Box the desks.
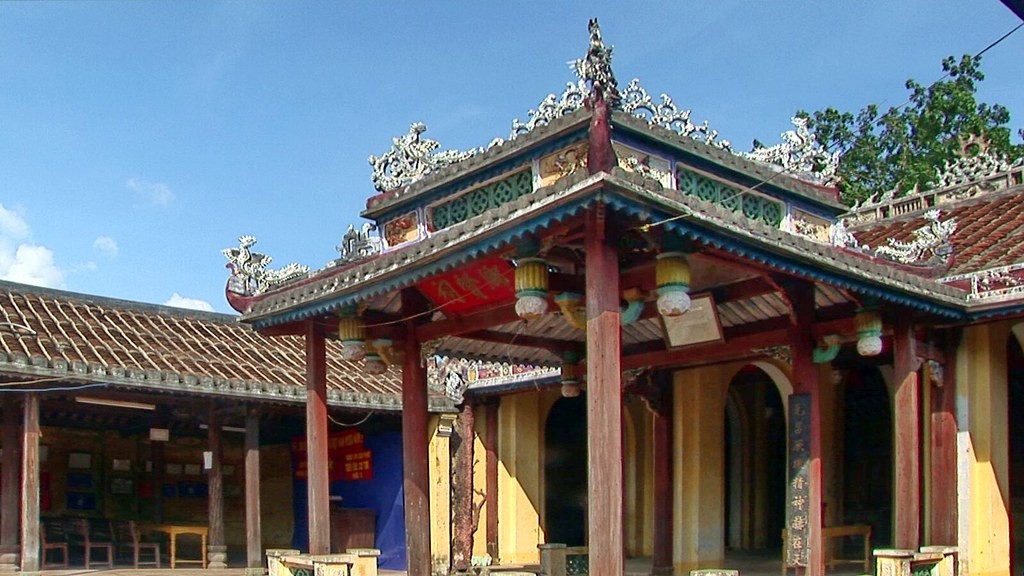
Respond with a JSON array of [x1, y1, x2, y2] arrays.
[[781, 525, 871, 576], [141, 523, 208, 569]]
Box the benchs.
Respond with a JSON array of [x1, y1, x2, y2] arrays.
[[40, 515, 160, 569]]
